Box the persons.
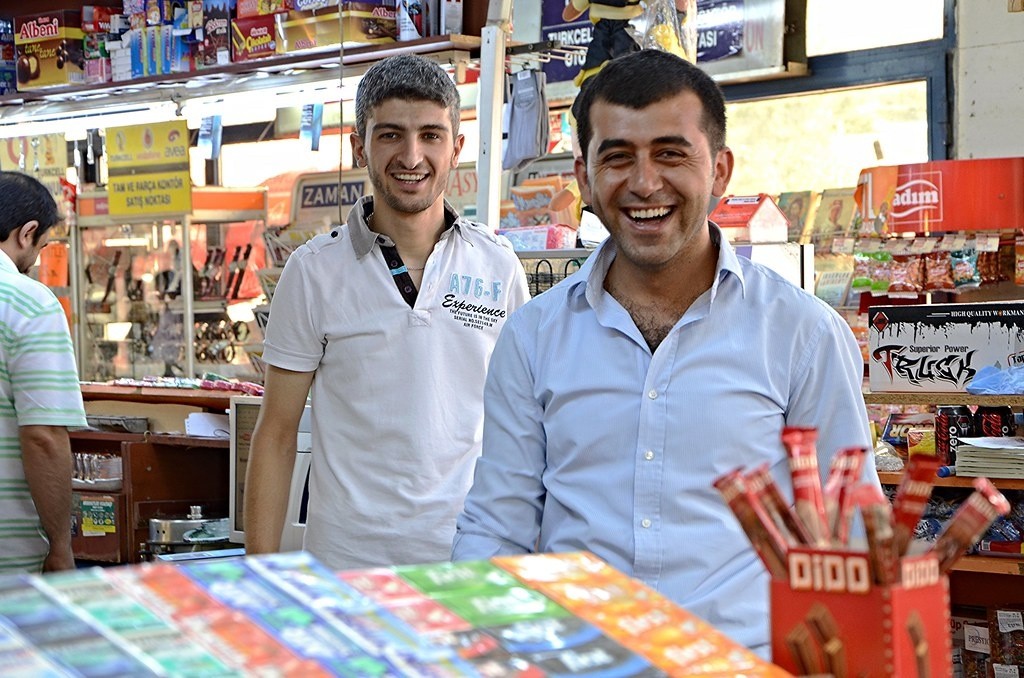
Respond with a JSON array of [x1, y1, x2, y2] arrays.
[[242, 51, 531, 571], [450, 48, 894, 660], [0, 171, 87, 574]]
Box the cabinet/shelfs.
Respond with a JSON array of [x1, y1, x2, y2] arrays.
[[506, 243, 814, 303], [65, 430, 224, 565], [0, 36, 530, 434], [849, 159, 1023, 677]]
